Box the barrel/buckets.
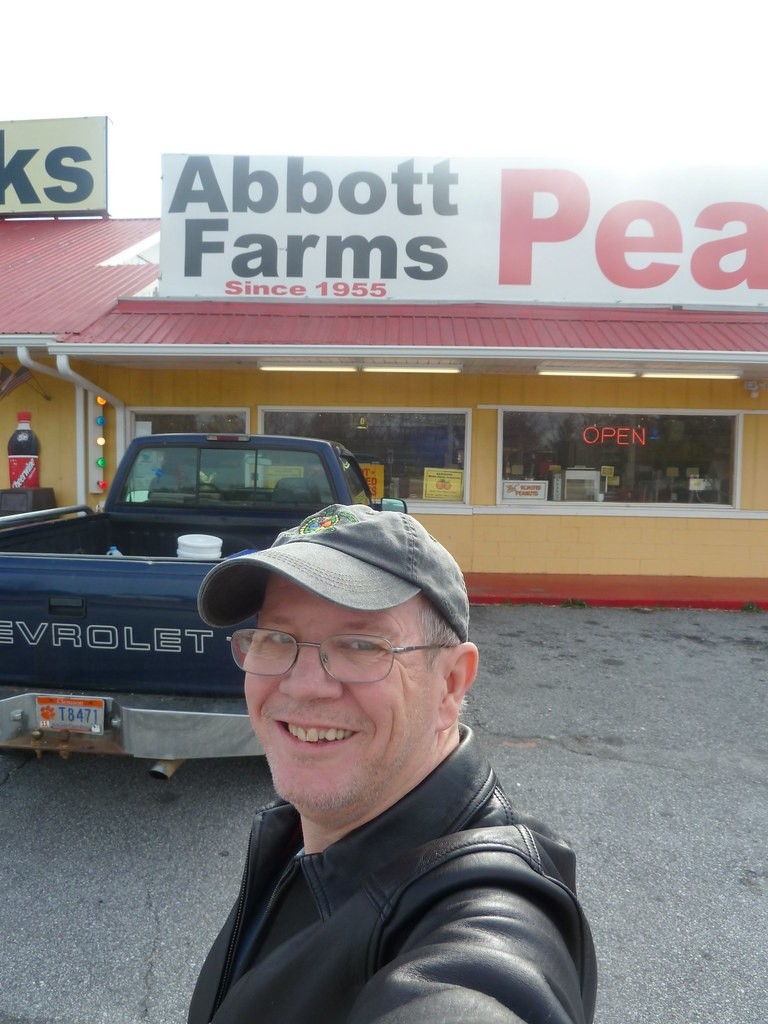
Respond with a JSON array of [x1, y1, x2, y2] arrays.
[[176, 534, 223, 558]]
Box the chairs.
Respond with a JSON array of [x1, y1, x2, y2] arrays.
[[143, 476, 193, 503], [271, 477, 322, 504]]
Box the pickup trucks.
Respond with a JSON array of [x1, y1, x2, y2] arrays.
[[0, 432, 409, 782]]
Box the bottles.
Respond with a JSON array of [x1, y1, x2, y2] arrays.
[[8, 412, 40, 488], [106, 546, 123, 556]]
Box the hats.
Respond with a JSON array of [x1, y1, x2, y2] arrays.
[[196, 503, 470, 644]]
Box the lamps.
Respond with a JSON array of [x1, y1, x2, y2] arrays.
[[743, 380, 760, 398]]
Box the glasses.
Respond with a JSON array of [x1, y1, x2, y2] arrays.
[[230, 626, 459, 684]]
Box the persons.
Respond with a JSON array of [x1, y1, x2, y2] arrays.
[[187, 504, 598, 1024]]
